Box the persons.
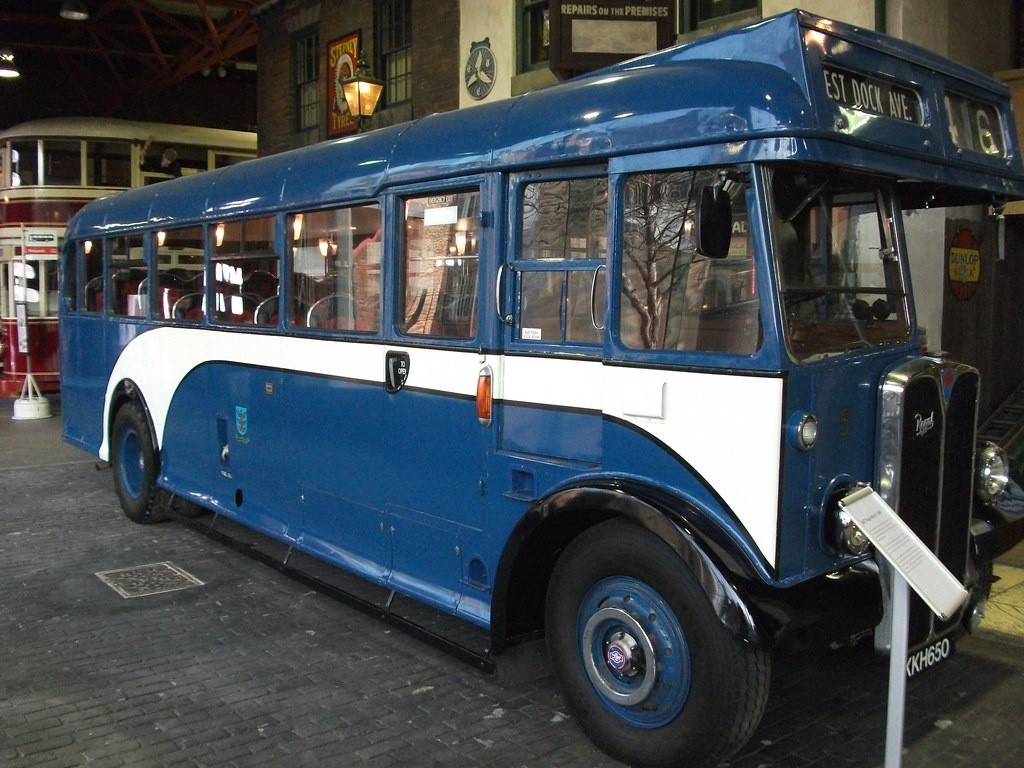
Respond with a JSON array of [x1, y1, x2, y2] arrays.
[[336, 63, 351, 100], [139, 149, 184, 185]]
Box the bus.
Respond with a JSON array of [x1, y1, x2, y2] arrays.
[[58, 8, 1022, 766], [0, 114, 257, 394]]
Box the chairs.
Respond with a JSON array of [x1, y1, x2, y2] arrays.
[[87, 264, 478, 340]]
